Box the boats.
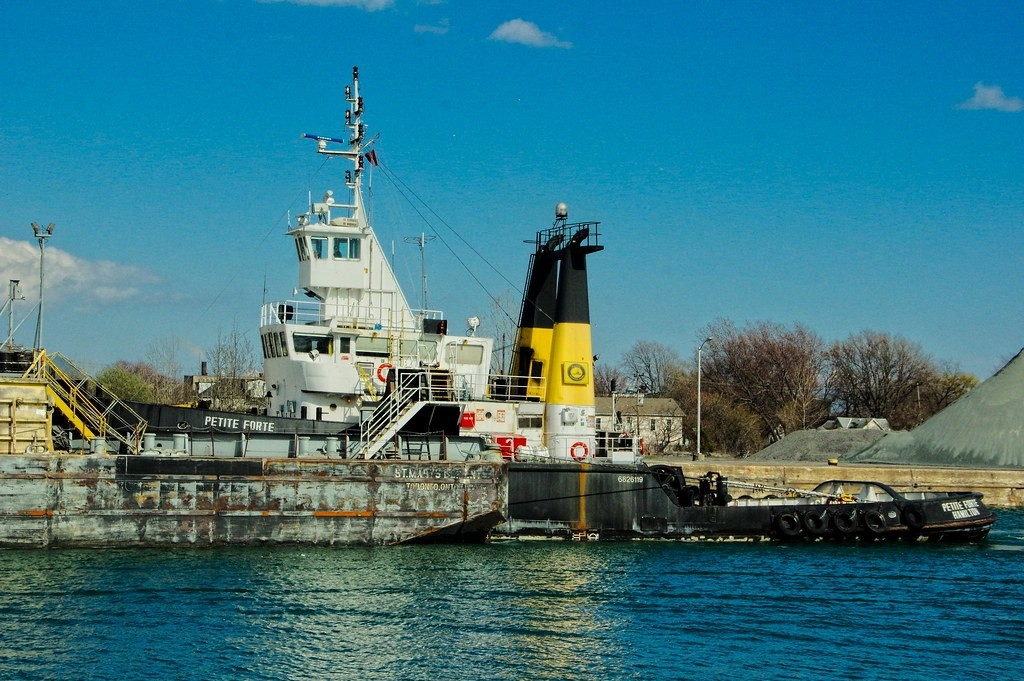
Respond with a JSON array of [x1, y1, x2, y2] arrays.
[[1, 67, 998, 547]]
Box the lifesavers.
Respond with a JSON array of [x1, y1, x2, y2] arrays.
[[377, 363, 393, 383], [773, 501, 928, 539], [570, 442, 588, 462], [640, 437, 645, 456]]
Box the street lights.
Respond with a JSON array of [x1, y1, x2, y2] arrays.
[[693, 337, 712, 460]]
